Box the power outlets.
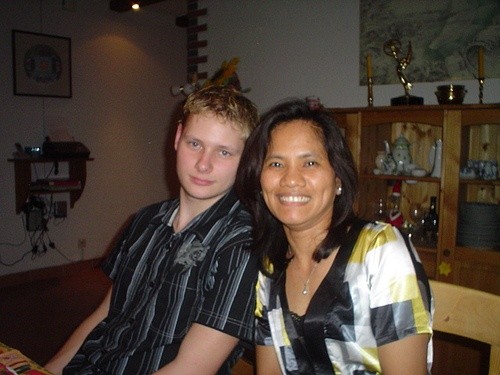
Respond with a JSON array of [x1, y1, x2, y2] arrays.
[[79, 239, 87, 247]]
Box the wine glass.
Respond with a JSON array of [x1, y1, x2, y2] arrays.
[[409, 203, 423, 226]]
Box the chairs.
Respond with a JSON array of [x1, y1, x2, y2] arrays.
[[429, 280, 500, 375]]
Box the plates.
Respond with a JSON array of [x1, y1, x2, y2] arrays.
[[457, 202, 500, 248]]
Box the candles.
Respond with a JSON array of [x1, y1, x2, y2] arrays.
[[479, 47, 484, 78], [367, 55, 372, 78]]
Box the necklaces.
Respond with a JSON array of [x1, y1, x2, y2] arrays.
[[291, 261, 319, 296]]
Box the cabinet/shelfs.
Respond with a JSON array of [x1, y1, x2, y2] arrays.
[[326, 103, 500, 375], [8, 158, 97, 214]]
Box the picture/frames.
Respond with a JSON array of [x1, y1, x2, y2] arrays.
[[12, 28, 72, 99]]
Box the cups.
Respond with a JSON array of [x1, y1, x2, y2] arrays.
[[460, 159, 498, 180], [373, 141, 427, 177]]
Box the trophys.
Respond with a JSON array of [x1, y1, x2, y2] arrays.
[[384, 38, 424, 106]]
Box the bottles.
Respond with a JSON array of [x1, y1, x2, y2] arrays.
[[375, 197, 388, 223], [423, 196, 438, 245], [431, 139, 441, 178]]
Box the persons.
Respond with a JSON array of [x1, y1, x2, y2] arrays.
[[239, 96, 435, 375], [45, 84, 256, 375]]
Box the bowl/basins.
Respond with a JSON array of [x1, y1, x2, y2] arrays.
[[435, 85, 468, 104]]
